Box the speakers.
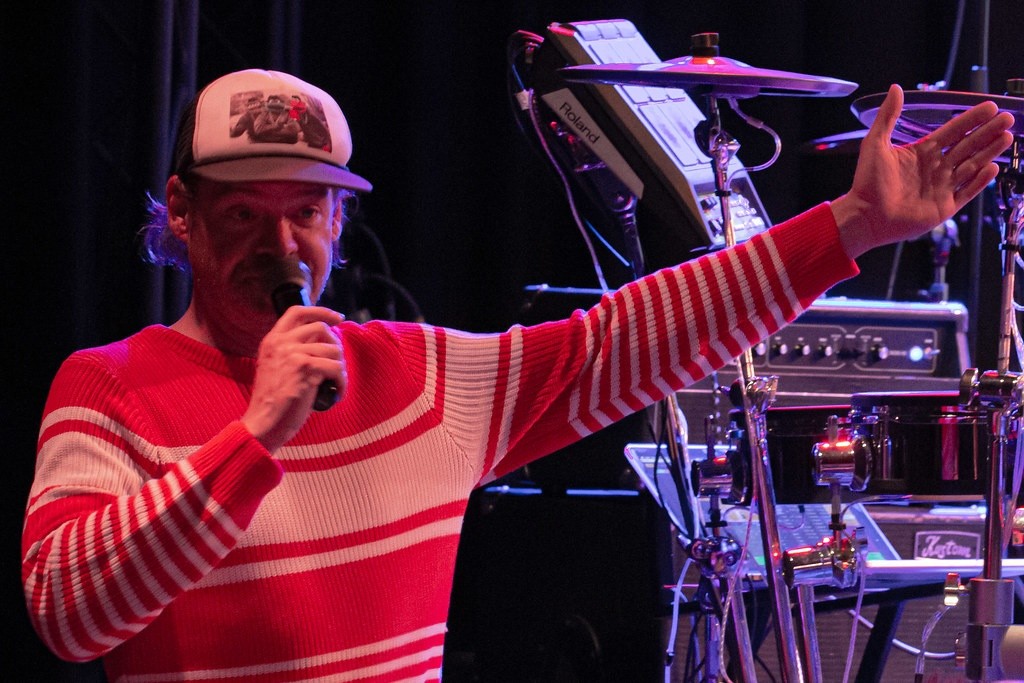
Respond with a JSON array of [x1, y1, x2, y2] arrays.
[[442, 484, 668, 683]]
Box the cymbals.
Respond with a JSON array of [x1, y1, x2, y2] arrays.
[[849, 91, 1024, 164], [549, 56, 860, 99], [797, 128, 869, 155]]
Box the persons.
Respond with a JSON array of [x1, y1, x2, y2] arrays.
[[21, 69, 1015, 683]]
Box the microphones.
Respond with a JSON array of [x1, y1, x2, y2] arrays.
[[264, 257, 338, 413]]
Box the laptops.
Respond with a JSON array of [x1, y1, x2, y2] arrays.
[[626, 440, 1024, 580]]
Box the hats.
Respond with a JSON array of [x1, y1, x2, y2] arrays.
[[181, 67, 373, 192]]
